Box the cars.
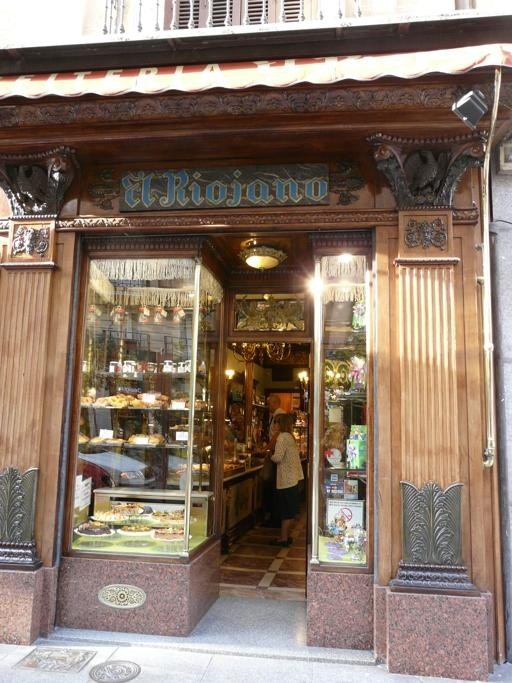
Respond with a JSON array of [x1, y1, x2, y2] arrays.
[[79, 434, 159, 490]]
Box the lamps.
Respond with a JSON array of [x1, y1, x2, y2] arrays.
[[448, 88, 488, 129], [238, 246, 288, 273], [65, 250, 231, 563]]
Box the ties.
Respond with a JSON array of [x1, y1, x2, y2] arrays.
[[269, 415, 274, 427]]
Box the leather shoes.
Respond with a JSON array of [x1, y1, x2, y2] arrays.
[[259, 520, 281, 528], [288, 537, 292, 544], [270, 539, 289, 548]]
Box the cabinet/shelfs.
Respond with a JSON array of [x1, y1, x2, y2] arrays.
[[167, 457, 307, 549], [311, 249, 374, 574]]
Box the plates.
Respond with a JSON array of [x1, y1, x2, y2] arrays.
[[73, 528, 115, 538], [128, 405, 160, 409], [88, 442, 124, 447], [123, 442, 159, 448], [153, 536, 191, 543], [116, 529, 153, 536], [166, 406, 197, 411], [90, 515, 129, 525], [148, 513, 195, 526], [92, 403, 123, 409]]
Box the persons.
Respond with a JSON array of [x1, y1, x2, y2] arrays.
[[258, 395, 287, 453], [270, 415, 305, 546], [254, 413, 284, 528]]
